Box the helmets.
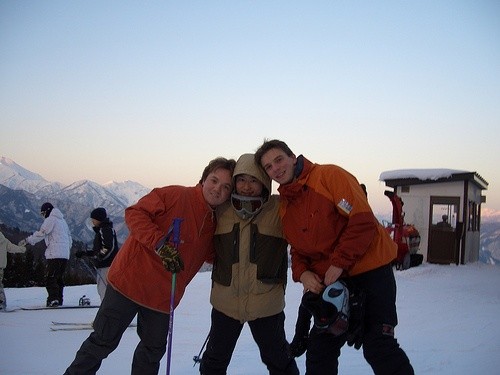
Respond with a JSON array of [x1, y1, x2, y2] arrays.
[[302, 279, 350, 331], [41, 203, 54, 212]]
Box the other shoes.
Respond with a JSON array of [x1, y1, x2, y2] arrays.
[[45, 300, 59, 307]]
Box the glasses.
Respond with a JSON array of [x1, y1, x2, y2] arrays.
[[230, 193, 263, 215]]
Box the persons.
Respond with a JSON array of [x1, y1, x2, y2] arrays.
[[65, 159, 237, 375], [255, 139, 415, 375], [18, 203, 72, 306], [0, 232, 26, 310], [437, 214, 451, 226], [197, 154, 301, 375], [76, 208, 119, 301]]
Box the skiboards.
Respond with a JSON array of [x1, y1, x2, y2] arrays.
[[16, 304, 101, 311], [49, 320, 138, 331]]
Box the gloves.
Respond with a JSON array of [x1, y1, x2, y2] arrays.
[[346, 293, 370, 349], [75, 251, 84, 259], [18, 239, 27, 247], [91, 255, 99, 265], [289, 323, 309, 356], [155, 245, 184, 273]]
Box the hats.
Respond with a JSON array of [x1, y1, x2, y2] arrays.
[[91, 207, 106, 221]]
